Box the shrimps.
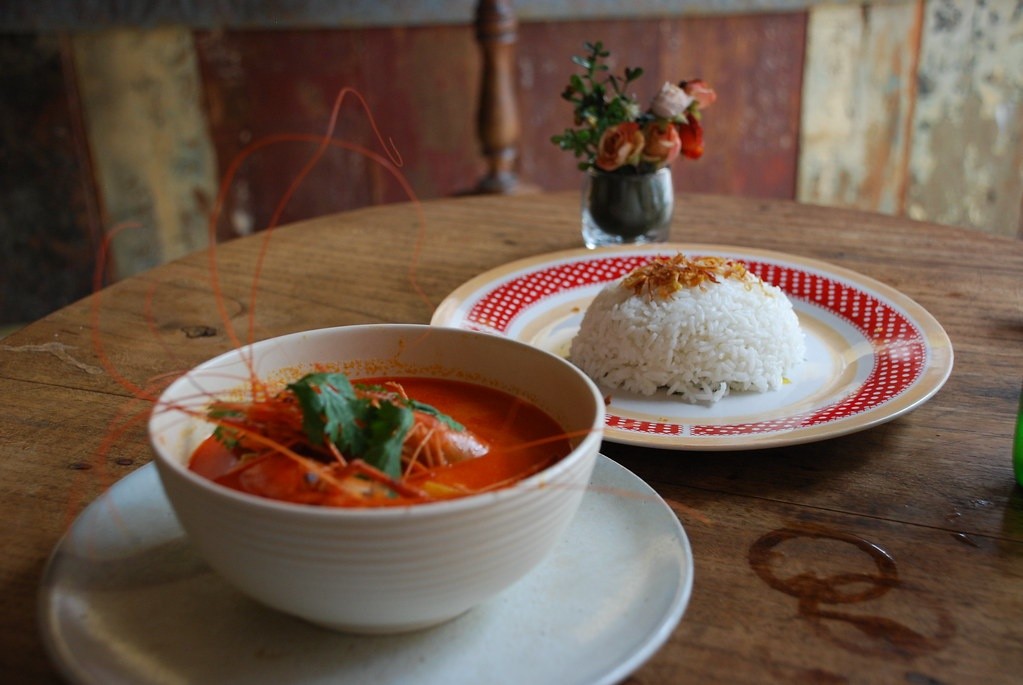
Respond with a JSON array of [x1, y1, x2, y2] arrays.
[[234, 384, 499, 507]]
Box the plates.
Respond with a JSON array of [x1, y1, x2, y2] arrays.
[[34, 434, 695, 685], [425, 241, 955, 451]]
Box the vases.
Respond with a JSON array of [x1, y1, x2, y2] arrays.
[[579, 166, 675, 251]]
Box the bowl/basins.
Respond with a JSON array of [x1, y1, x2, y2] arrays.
[[151, 322, 605, 635]]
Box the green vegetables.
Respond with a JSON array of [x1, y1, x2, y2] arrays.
[[208, 371, 466, 497]]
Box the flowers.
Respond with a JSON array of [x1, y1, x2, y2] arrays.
[[547, 38, 714, 231]]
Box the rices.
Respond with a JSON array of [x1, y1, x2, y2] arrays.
[[568, 248, 809, 406]]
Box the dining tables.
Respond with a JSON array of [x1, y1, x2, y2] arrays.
[[0, 184, 1022, 685]]
